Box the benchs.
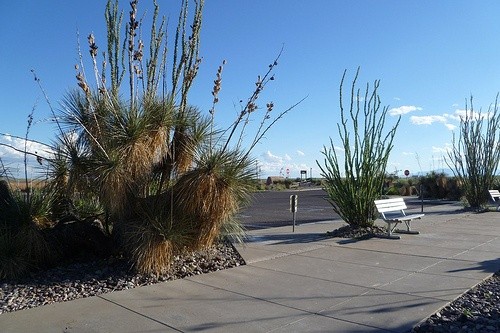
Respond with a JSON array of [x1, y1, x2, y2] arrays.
[[488, 189, 500, 210], [374, 197, 425, 239]]
